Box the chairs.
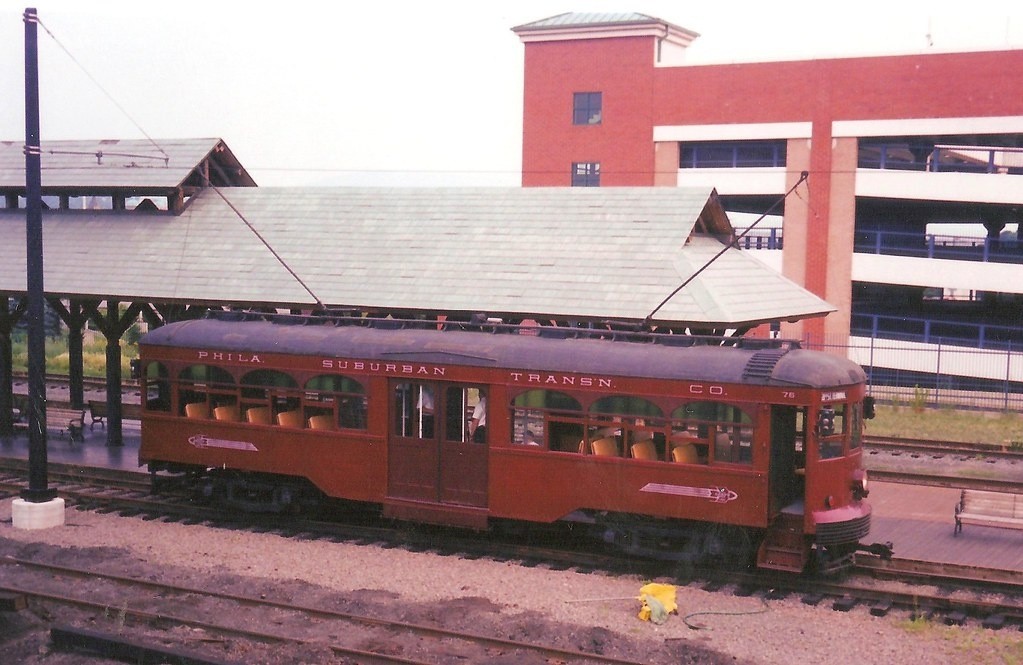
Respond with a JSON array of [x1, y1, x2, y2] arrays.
[[184, 402, 336, 428], [564, 426, 731, 464]]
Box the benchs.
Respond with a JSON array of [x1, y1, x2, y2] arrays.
[[12, 393, 30, 424], [87, 400, 142, 430], [953, 487, 1023, 535]]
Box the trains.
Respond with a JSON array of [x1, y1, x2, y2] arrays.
[[136, 309, 897, 587]]
